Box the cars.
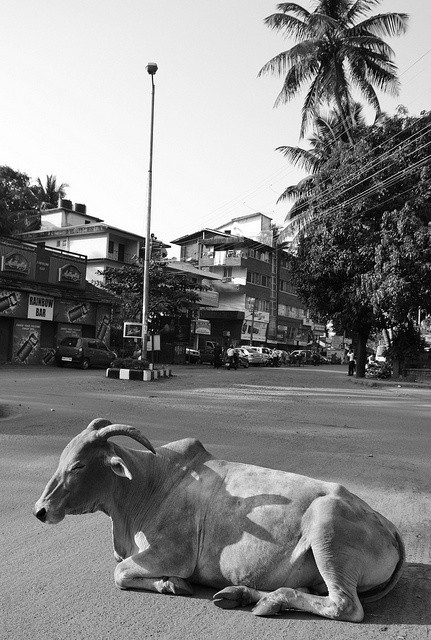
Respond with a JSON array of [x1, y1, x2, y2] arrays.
[[232, 350, 248, 367], [235, 348, 263, 366]]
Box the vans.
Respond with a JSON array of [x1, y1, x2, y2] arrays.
[[290, 350, 313, 358], [241, 346, 278, 363], [56, 336, 117, 369]]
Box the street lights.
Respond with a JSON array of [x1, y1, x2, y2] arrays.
[[141, 62, 158, 361]]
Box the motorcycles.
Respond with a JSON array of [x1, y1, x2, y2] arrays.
[[265, 357, 281, 367], [224, 355, 239, 370]]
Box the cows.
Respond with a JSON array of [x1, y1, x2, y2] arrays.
[[30, 416, 406, 624]]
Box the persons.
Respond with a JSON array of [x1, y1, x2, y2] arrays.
[[272, 348, 279, 366], [366, 355, 374, 368], [227, 344, 238, 366], [282, 351, 287, 365], [348, 349, 355, 376], [214, 343, 222, 368], [133, 344, 143, 360]]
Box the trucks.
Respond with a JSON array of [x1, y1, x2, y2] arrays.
[[196, 341, 223, 364]]
